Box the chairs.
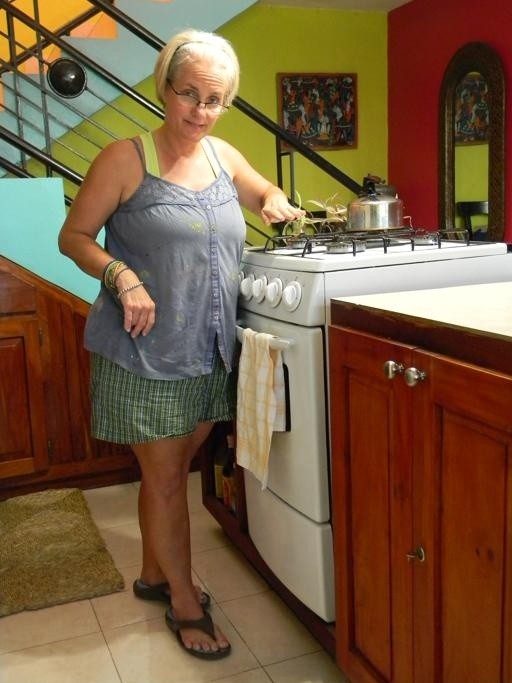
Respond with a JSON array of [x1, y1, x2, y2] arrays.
[[456, 201, 488, 241]]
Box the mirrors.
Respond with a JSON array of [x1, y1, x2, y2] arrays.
[[438, 40, 505, 243]]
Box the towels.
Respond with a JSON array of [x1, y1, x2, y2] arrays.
[[235, 328, 285, 489]]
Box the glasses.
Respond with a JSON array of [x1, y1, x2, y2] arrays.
[[166, 80, 232, 115]]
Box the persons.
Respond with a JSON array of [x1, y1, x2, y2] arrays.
[[53, 24, 309, 660]]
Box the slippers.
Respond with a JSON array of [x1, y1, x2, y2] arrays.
[[133, 576, 214, 608], [162, 602, 231, 661]]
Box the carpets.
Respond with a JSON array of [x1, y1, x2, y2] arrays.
[[0, 487, 125, 616]]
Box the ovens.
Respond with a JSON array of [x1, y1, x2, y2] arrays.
[[229, 309, 332, 626]]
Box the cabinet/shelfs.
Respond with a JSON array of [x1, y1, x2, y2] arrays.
[[0, 256, 144, 487], [201, 418, 247, 547], [327, 325, 512, 683]]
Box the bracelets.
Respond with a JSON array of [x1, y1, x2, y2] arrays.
[[117, 280, 148, 300], [101, 258, 130, 294]]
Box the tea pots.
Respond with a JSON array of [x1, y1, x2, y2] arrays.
[[347, 177, 405, 231]]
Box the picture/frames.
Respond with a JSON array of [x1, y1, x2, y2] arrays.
[[275, 72, 358, 152]]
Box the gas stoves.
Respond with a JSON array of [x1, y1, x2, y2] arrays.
[[235, 228, 509, 325]]
